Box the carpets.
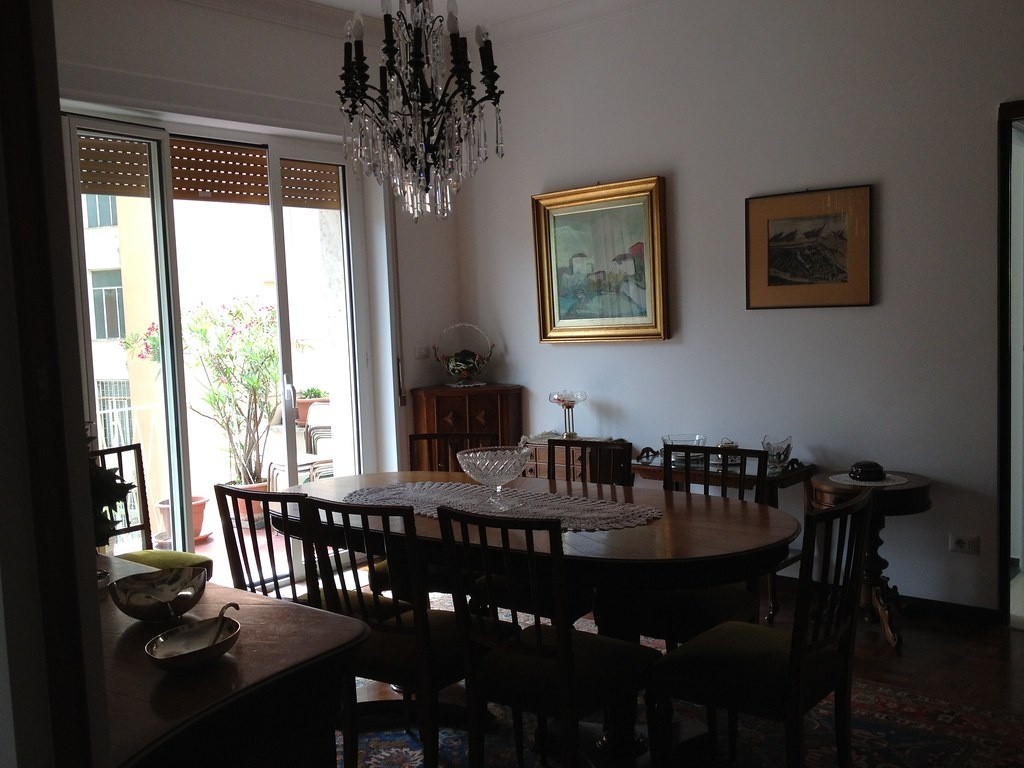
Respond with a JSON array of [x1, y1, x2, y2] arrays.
[[342, 677, 1024, 768]]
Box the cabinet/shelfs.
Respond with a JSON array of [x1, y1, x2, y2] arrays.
[[95, 554, 374, 768], [409, 381, 523, 470], [519, 442, 617, 483]]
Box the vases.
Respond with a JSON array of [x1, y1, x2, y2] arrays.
[[155, 495, 210, 538], [225, 476, 271, 515]]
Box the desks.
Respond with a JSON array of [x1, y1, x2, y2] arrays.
[[809, 470, 933, 648], [633, 453, 818, 510], [276, 471, 801, 768]]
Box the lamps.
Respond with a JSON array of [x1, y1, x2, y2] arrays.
[[334, 0, 505, 222]]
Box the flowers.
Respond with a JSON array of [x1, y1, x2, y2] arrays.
[[117, 292, 283, 484]]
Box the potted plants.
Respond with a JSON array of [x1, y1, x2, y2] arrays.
[[297, 386, 329, 422]]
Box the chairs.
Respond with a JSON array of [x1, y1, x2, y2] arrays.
[[215, 439, 884, 768]]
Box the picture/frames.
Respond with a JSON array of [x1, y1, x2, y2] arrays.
[[744, 183, 874, 310], [531, 175, 672, 344]]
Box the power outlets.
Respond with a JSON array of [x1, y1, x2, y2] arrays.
[[948, 534, 980, 556]]
